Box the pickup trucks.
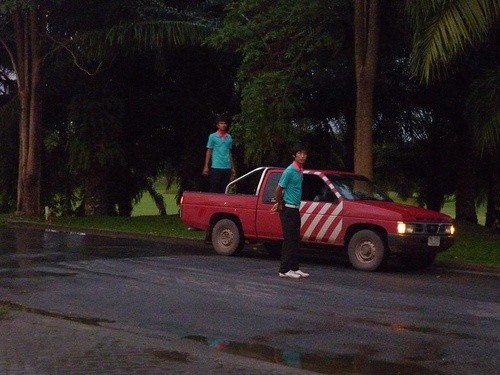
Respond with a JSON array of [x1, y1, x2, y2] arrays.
[[179, 167, 459, 273]]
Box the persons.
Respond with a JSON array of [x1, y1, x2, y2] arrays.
[[274, 145, 311, 279], [202, 116, 236, 193]]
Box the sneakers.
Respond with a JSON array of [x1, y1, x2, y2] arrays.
[[278, 269, 301, 278], [294, 270, 309, 277]]
